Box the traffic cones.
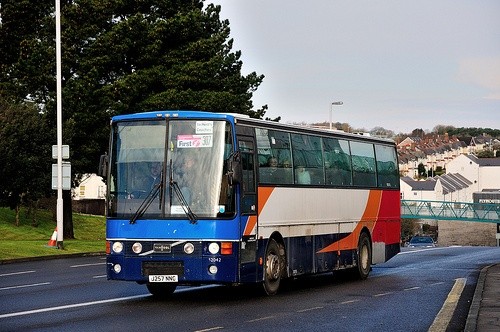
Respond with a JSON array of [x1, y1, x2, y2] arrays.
[[47, 227, 60, 249]]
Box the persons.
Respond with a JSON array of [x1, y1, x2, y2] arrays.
[[259, 156, 346, 185], [127, 162, 161, 200]]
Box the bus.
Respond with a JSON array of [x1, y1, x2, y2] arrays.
[[106, 110, 401, 300]]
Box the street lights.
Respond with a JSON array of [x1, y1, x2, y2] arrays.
[[330, 101, 344, 130]]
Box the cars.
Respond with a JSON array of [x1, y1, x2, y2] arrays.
[[406, 236, 435, 248]]
